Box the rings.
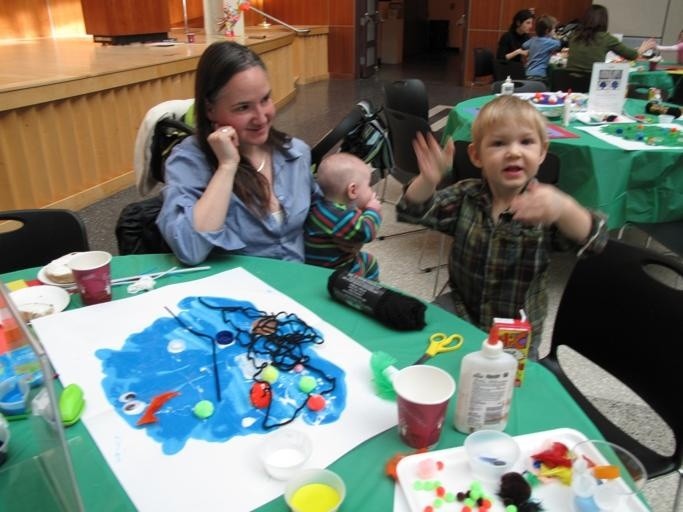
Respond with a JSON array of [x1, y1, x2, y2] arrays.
[[221, 128, 229, 133]]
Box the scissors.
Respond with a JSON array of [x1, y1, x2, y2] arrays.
[[413, 332, 464, 366]]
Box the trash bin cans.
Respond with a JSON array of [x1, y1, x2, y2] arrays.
[[428, 19, 450, 53]]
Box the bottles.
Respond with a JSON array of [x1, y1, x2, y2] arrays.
[[449, 328, 516, 433], [562, 90, 572, 127], [647, 87, 661, 103], [500, 77, 514, 95]]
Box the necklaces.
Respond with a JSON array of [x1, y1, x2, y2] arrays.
[[256, 146, 267, 175]]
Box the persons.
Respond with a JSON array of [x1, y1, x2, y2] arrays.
[[156, 40, 324, 267], [395, 95, 612, 363], [493, 3, 683, 93], [303, 152, 383, 284]]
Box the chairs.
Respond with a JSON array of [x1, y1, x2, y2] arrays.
[[114, 192, 164, 255], [536, 237, 683, 512], [0, 208, 89, 279]]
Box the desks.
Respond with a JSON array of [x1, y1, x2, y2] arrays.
[[386, 40, 683, 244], [0, 256, 646, 512]]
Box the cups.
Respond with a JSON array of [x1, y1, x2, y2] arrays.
[[658, 114, 674, 129], [283, 464, 344, 512], [69, 251, 112, 307], [647, 59, 655, 71], [185, 32, 195, 43], [463, 427, 520, 477], [394, 362, 454, 449], [567, 441, 648, 511], [0, 374, 26, 464]]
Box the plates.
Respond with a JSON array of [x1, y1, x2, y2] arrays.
[[9, 286, 70, 325], [36, 252, 73, 288], [529, 91, 563, 108]]
